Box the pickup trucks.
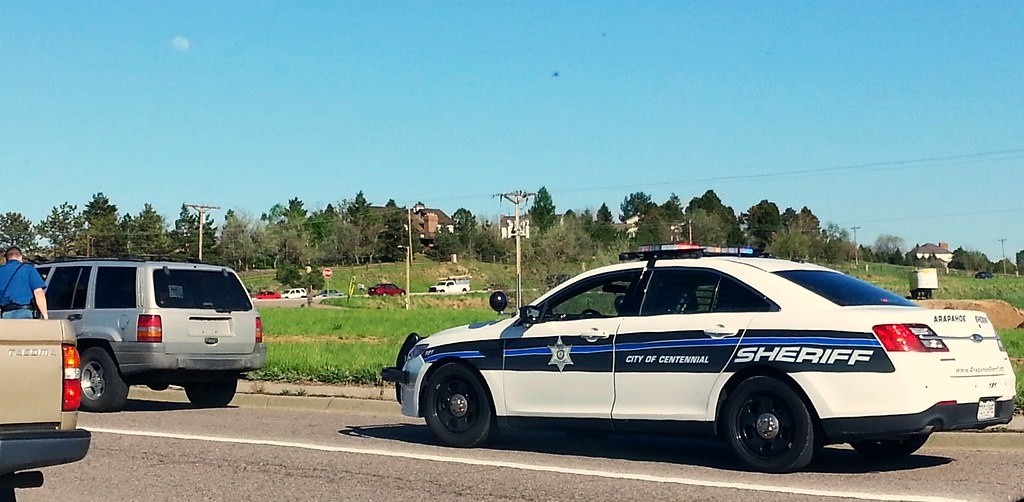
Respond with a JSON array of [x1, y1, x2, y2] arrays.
[[0, 317, 92, 501]]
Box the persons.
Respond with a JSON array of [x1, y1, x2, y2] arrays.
[[0, 248, 51, 320]]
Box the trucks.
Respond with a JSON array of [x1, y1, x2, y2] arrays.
[[430, 276, 470, 294]]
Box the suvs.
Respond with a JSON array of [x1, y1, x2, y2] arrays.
[[30, 259, 266, 409]]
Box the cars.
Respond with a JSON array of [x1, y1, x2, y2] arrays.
[[383, 243, 1017, 475], [250, 290, 281, 299], [282, 287, 309, 298], [975, 270, 993, 279], [368, 283, 406, 296], [544, 274, 571, 289], [483, 283, 509, 290], [316, 288, 344, 300]]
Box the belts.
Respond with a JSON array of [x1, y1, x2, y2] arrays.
[[1, 304, 33, 312]]
